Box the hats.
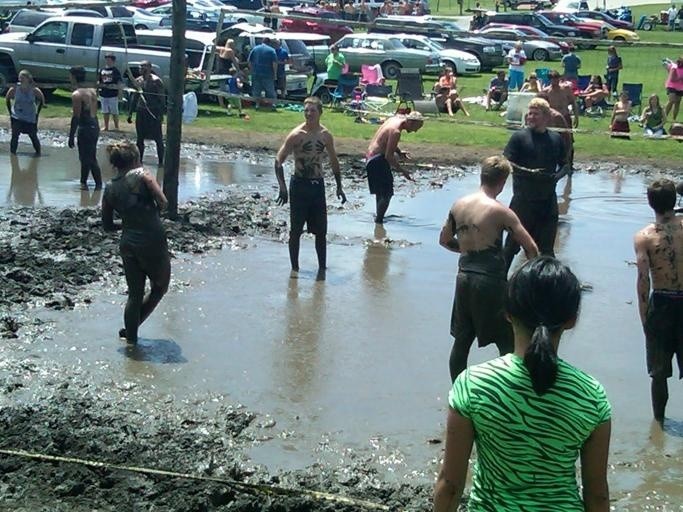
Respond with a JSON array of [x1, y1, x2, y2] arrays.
[[329, 45, 338, 49], [498, 70, 505, 76], [406, 110, 429, 121], [515, 40, 524, 48]]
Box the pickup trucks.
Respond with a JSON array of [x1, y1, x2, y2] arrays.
[[0, 17, 206, 93], [203, 36, 313, 92]]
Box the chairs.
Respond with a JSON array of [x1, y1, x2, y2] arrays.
[[326, 87, 346, 108], [622, 84, 642, 116], [575, 74, 592, 91]]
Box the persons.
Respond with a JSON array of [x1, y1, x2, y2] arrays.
[[497, 98, 572, 271], [485, 71, 510, 112], [439, 68, 470, 117], [580, 1, 636, 27], [523, 106, 573, 176], [68, 66, 102, 191], [102, 141, 171, 345], [228, 67, 247, 117], [579, 74, 609, 117], [260, 0, 272, 29], [380, 0, 430, 17], [215, 39, 239, 109], [127, 61, 166, 165], [6, 69, 44, 154], [267, 38, 289, 101], [433, 81, 460, 101], [357, 1, 370, 30], [508, 41, 527, 93], [433, 254, 612, 512], [606, 46, 623, 101], [495, 1, 500, 13], [275, 97, 347, 270], [267, 0, 283, 30], [366, 111, 424, 224], [536, 71, 580, 143], [667, 5, 678, 31], [560, 46, 581, 79], [664, 58, 683, 121], [344, 1, 355, 32], [520, 72, 544, 93], [609, 90, 632, 139], [97, 55, 123, 131], [324, 45, 346, 80], [640, 94, 667, 138], [471, 16, 479, 30], [438, 154, 539, 387], [632, 180, 683, 419], [248, 37, 277, 111], [474, 12, 484, 28], [675, 5, 683, 31]]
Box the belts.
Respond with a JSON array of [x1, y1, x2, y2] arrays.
[[295, 177, 323, 185]]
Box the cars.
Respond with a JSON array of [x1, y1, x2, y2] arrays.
[[391, 34, 482, 75], [234, 0, 640, 71], [306, 33, 446, 81], [1, 0, 237, 33]]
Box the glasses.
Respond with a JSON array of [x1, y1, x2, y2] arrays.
[[529, 76, 536, 79]]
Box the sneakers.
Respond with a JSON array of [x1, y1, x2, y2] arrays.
[[239, 112, 244, 116], [226, 112, 232, 116], [254, 103, 259, 111], [272, 103, 276, 110]]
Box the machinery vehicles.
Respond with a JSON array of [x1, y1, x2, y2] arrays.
[[642, 15, 682, 31]]
